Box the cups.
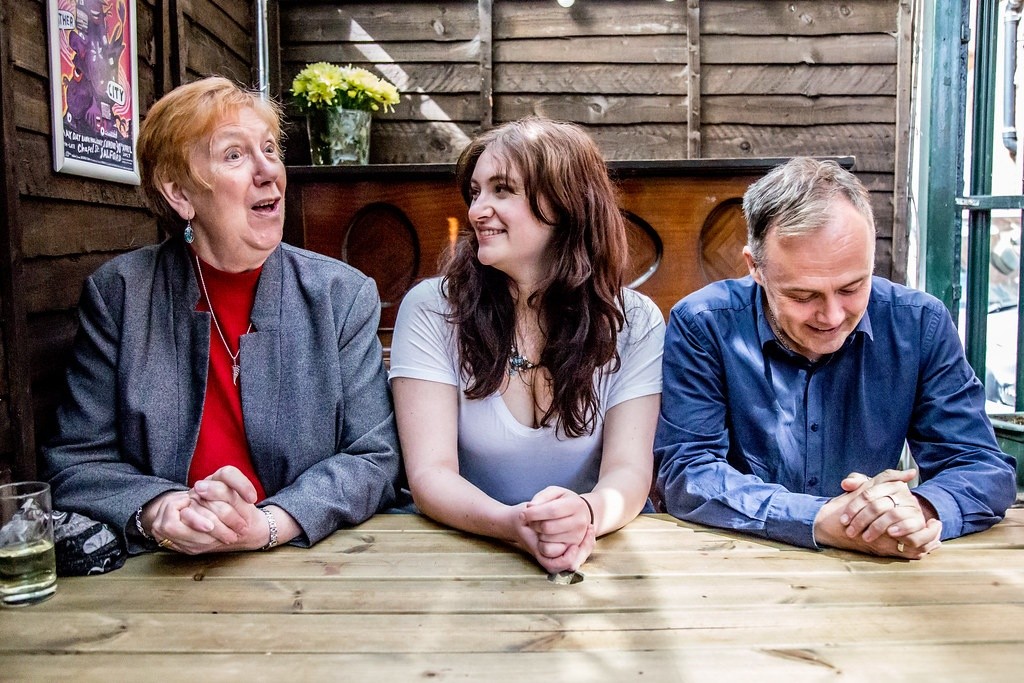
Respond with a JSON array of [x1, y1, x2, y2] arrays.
[[0, 481, 57, 608]]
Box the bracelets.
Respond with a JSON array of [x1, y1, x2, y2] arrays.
[[579, 494, 595, 525], [260, 506, 278, 552]]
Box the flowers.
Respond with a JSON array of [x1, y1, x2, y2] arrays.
[[290, 61, 401, 165]]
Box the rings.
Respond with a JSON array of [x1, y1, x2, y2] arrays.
[[897, 542, 905, 553], [885, 494, 900, 508], [159, 539, 173, 548]]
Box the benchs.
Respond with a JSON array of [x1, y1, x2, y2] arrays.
[[281, 156, 855, 372]]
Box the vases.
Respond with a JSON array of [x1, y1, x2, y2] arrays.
[[307, 109, 372, 165]]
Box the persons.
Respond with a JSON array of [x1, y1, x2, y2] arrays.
[[389, 116, 668, 575], [45, 74, 400, 556], [653, 154, 1018, 561]]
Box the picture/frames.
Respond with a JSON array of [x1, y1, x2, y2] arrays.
[[46, 0, 141, 185]]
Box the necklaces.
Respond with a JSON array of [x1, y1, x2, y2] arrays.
[[506, 345, 534, 379], [194, 253, 253, 386], [768, 310, 789, 346]]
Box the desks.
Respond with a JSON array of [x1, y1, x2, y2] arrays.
[[0, 504, 1024, 683]]
